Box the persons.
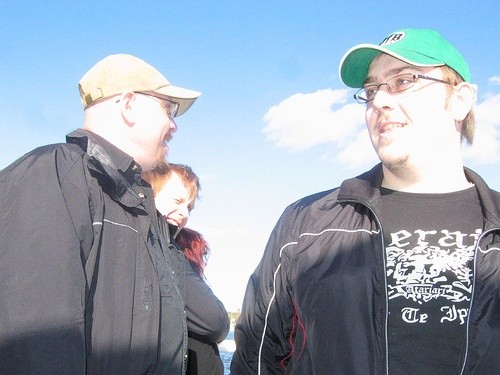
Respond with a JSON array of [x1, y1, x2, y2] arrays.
[[0, 53, 229, 375], [229, 29, 500, 375]]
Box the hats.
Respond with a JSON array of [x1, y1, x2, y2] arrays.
[[339, 29, 473, 88], [78, 53, 202, 118]]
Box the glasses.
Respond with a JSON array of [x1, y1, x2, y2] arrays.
[[115, 91, 181, 118], [353, 73, 452, 102]]
